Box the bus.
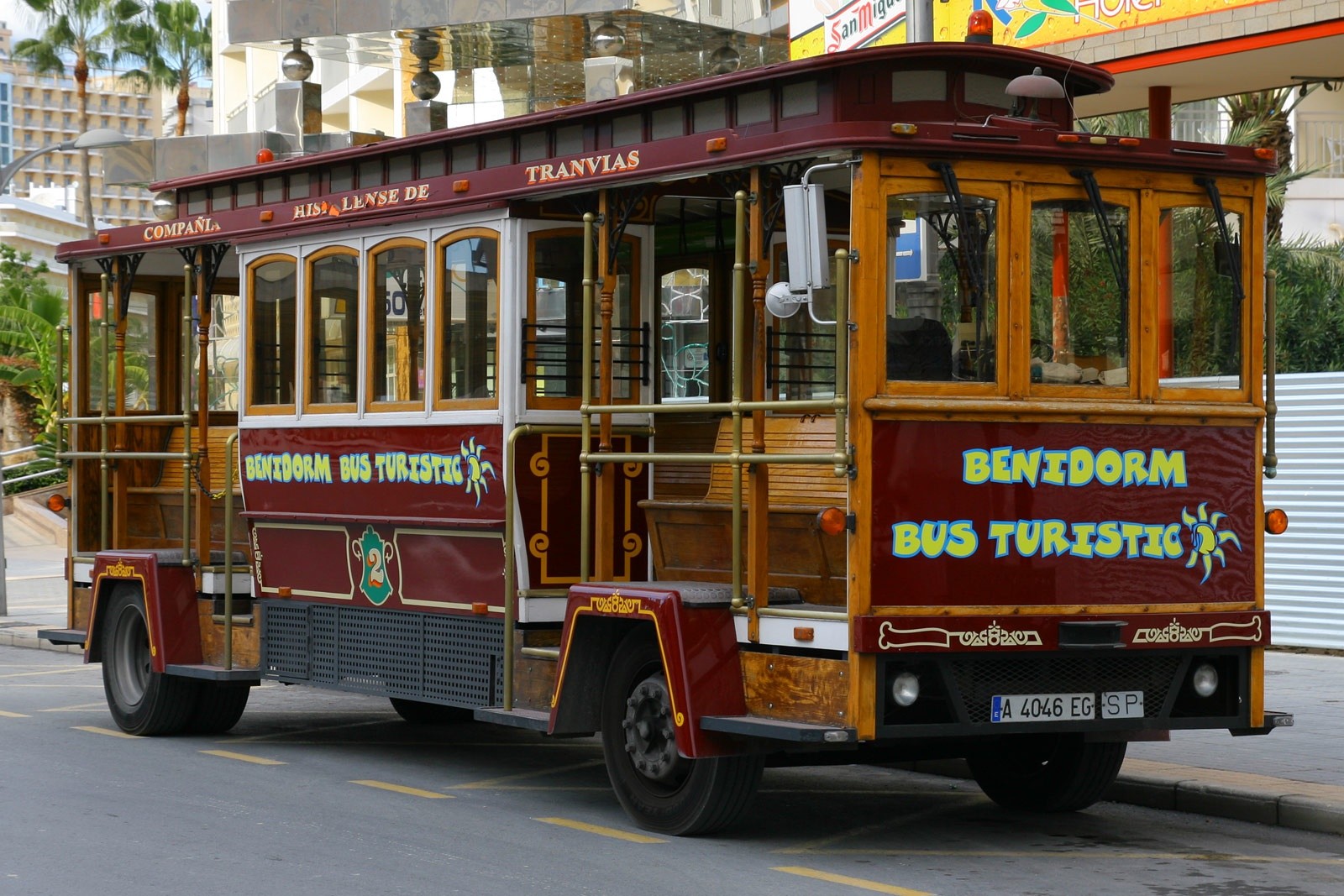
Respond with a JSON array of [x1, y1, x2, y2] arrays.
[[38, 10, 1295, 835]]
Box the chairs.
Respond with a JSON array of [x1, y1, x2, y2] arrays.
[[886, 314, 956, 380]]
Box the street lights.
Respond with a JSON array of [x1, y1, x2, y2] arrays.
[[1, 127, 132, 617]]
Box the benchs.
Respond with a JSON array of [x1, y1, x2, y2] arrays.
[[104, 423, 250, 547], [630, 414, 848, 598]]
